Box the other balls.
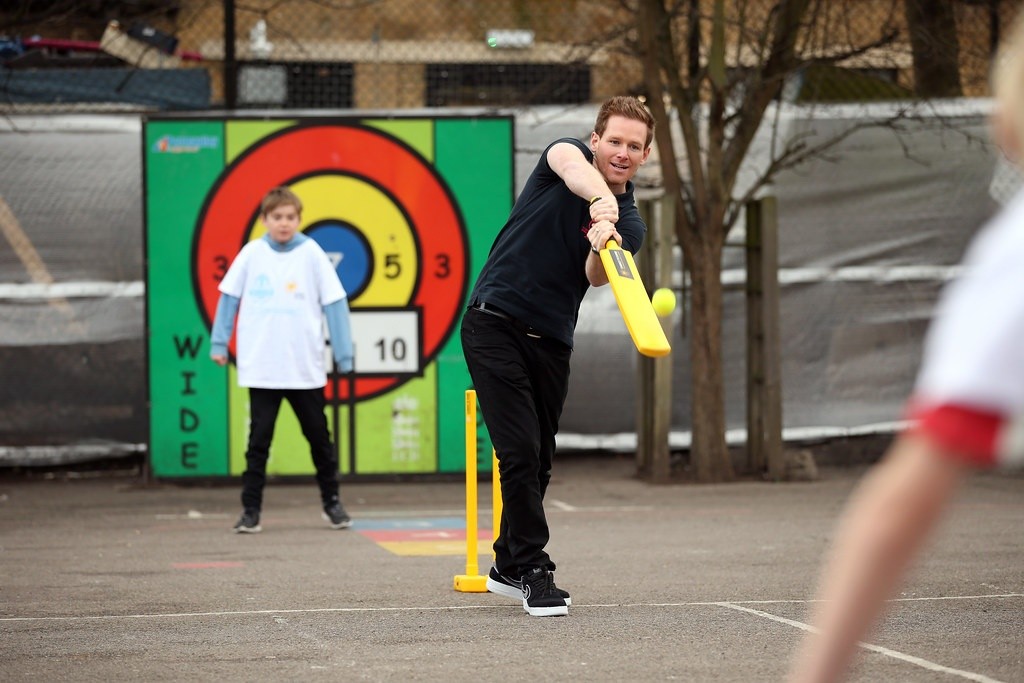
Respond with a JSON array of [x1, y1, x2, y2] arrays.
[[651, 287, 677, 317]]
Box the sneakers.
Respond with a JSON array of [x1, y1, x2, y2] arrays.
[[321, 501, 352, 529], [233, 511, 262, 532], [521, 563, 568, 616], [486, 564, 572, 606]]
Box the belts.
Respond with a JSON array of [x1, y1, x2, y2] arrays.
[[472, 301, 550, 339]]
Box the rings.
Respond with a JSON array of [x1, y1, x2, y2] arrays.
[[596, 228, 599, 232]]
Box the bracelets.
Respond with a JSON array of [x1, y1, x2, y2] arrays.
[[592, 247, 600, 256]]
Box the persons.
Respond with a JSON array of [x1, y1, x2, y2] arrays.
[[208, 188, 353, 534], [459, 97, 655, 617], [793, 13, 1024, 682]]
